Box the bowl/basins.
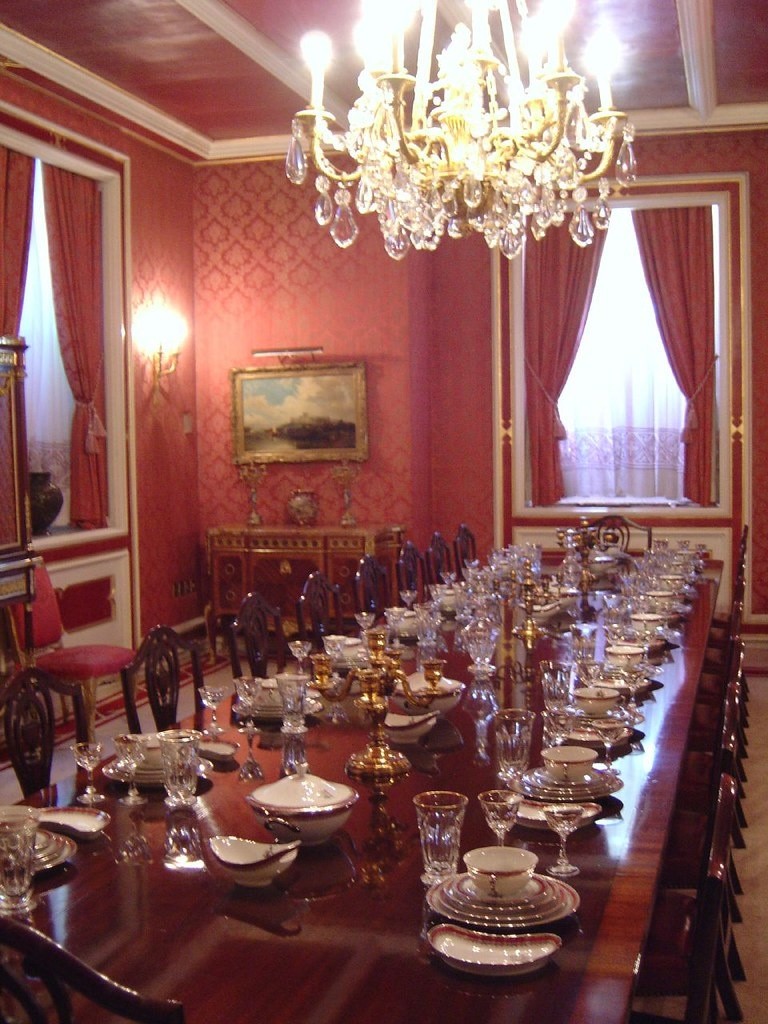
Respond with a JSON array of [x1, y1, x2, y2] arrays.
[[464, 846, 537, 895], [572, 686, 619, 715], [248, 760, 359, 848], [631, 613, 662, 633], [541, 747, 597, 779], [647, 590, 673, 603], [210, 836, 300, 886], [395, 666, 466, 716], [605, 647, 643, 669], [661, 575, 684, 586], [384, 713, 439, 743]]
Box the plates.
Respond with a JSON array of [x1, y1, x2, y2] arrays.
[[103, 759, 212, 782], [430, 924, 561, 974], [31, 830, 76, 871], [37, 807, 110, 837], [426, 874, 582, 928], [197, 738, 239, 760], [512, 799, 601, 828], [505, 771, 624, 801]]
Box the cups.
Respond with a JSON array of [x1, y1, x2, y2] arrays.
[[571, 623, 596, 666], [158, 730, 202, 805], [541, 661, 572, 711], [602, 592, 628, 638], [275, 674, 310, 731], [161, 812, 205, 873], [415, 790, 468, 881], [0, 806, 39, 918], [494, 707, 536, 773]]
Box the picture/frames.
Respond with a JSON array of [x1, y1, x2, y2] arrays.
[[229, 359, 371, 465]]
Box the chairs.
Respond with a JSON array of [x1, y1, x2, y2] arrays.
[[120, 625, 205, 735], [352, 554, 391, 621], [393, 540, 426, 607], [226, 590, 287, 684], [629, 523, 750, 1024], [556, 513, 652, 550], [0, 666, 89, 799], [296, 571, 343, 646], [425, 531, 451, 587], [0, 916, 186, 1024], [452, 523, 475, 582], [3, 555, 138, 742]]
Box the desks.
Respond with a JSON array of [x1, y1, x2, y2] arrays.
[[0, 547, 723, 1024]]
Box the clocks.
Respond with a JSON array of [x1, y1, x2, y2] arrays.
[[286, 1, 641, 261]]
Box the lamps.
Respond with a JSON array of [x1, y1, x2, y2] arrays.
[[132, 285, 190, 404], [252, 346, 323, 364]]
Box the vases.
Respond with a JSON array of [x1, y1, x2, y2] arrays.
[[30, 471, 63, 537]]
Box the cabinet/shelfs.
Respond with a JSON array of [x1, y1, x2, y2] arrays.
[[205, 525, 408, 660]]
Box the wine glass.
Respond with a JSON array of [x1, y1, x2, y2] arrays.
[[70, 742, 104, 804], [479, 791, 524, 846], [543, 804, 583, 877], [113, 807, 153, 866], [113, 735, 150, 804], [199, 540, 692, 769]]
